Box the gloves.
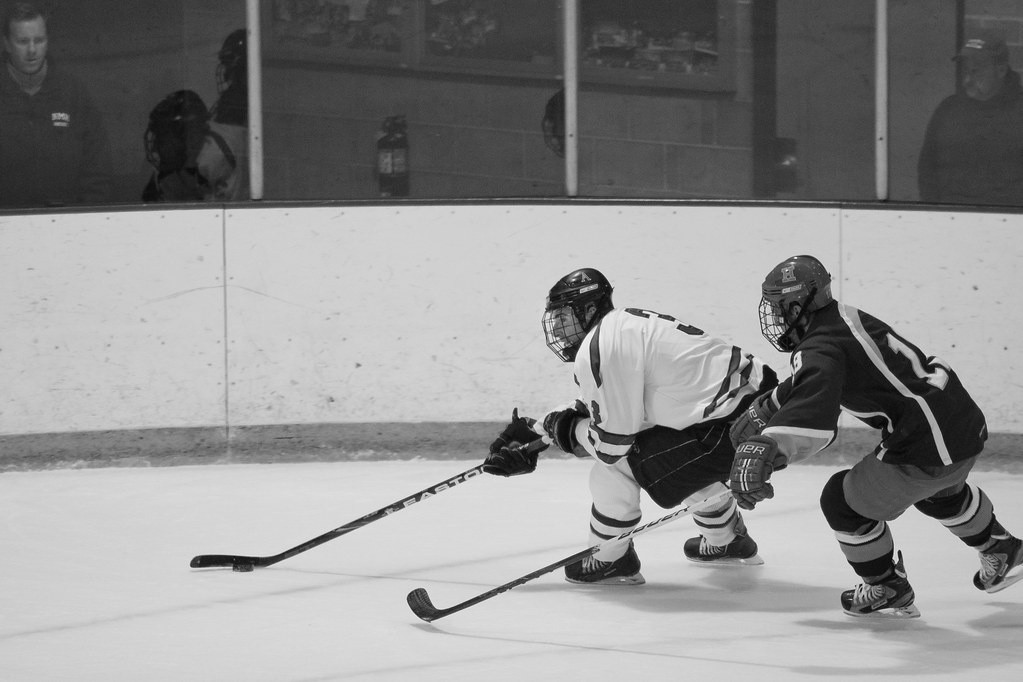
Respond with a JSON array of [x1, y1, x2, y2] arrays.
[[729, 391, 772, 451], [731, 438, 785, 510], [544, 408, 589, 458], [483, 407, 549, 477]]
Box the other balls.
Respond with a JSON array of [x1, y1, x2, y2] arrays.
[[232, 560, 254, 573]]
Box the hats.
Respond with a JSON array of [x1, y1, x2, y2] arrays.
[[951, 33, 1008, 60]]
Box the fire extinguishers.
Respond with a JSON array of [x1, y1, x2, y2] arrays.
[[375, 114, 408, 199]]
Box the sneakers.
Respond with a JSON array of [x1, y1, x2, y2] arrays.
[[840, 550, 920, 620], [972, 519, 1022, 593], [564, 538, 645, 587], [683, 511, 763, 567]]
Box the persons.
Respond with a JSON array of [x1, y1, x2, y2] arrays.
[[728, 256, 1023, 619], [1, 1, 114, 210], [483, 267, 780, 588], [916, 29, 1023, 212]]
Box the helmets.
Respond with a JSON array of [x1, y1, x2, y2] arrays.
[[542, 87, 563, 158], [758, 255, 833, 352], [144, 90, 211, 172], [542, 268, 615, 362], [214, 29, 247, 91]]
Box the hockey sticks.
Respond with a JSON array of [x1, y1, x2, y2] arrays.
[[405, 489, 734, 623], [187, 432, 557, 571]]
